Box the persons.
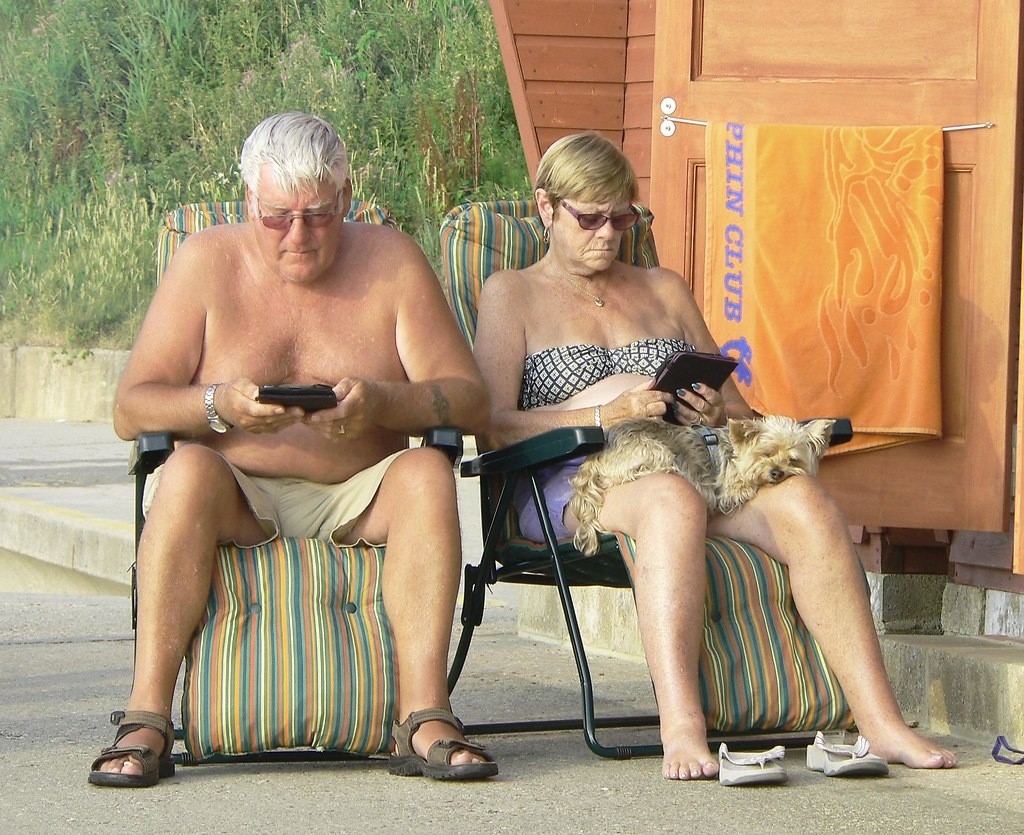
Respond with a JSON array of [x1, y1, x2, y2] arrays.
[[88, 111, 499, 788], [473, 133, 959, 781]]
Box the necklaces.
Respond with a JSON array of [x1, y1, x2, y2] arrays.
[[544, 257, 609, 307]]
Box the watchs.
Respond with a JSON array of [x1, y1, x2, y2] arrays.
[[204, 384, 234, 433]]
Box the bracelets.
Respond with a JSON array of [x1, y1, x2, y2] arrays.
[[594, 405, 602, 427]]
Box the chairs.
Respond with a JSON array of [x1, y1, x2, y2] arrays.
[[439, 200, 855, 761], [128, 204, 465, 766]]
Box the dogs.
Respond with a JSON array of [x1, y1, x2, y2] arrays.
[[566, 415, 837, 559]]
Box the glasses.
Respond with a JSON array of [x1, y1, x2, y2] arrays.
[[257, 192, 339, 229], [559, 198, 639, 230]]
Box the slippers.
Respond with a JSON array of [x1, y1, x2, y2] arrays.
[[719, 743, 786, 785], [807, 731, 889, 777]]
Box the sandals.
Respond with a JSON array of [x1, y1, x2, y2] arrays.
[[88, 711, 175, 787], [389, 708, 498, 780]]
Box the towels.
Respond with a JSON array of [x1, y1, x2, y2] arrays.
[[702, 122, 944, 459]]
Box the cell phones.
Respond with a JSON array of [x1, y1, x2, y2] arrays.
[[262, 385, 338, 412]]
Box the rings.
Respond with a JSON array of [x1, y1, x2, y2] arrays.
[[699, 417, 703, 423], [337, 425, 345, 435]]
